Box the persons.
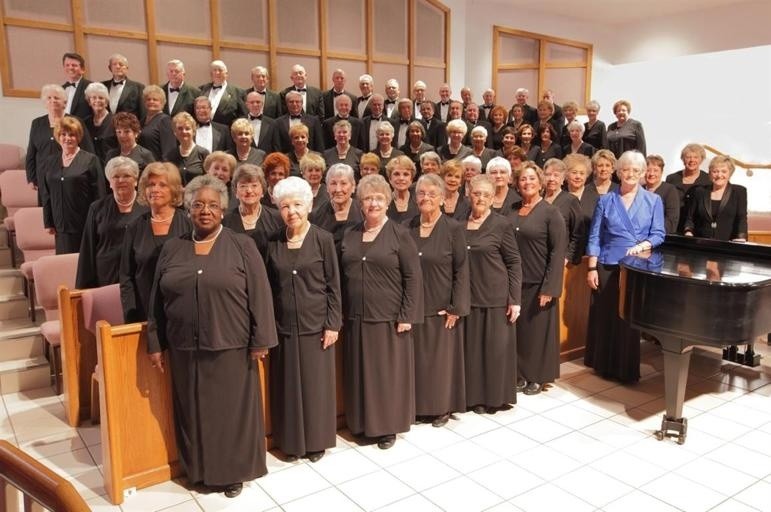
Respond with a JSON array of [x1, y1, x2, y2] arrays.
[[262, 176, 343, 462], [26, 52, 246, 258], [336, 174, 425, 449], [401, 173, 470, 427], [220, 64, 413, 273], [147, 175, 278, 497], [588, 148, 666, 384], [398, 79, 647, 265], [117, 160, 190, 323], [505, 160, 568, 394], [641, 143, 746, 241], [456, 174, 522, 414], [75, 155, 144, 292]]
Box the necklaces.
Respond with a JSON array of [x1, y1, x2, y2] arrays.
[[521, 197, 541, 207], [239, 202, 262, 224], [471, 209, 490, 222], [391, 190, 410, 206], [267, 187, 273, 199], [151, 215, 174, 222], [364, 216, 388, 232], [190, 223, 223, 244], [420, 211, 442, 228], [62, 147, 80, 160], [286, 220, 311, 242], [113, 190, 136, 206], [443, 191, 458, 207]]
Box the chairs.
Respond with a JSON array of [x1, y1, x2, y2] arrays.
[[82, 282, 125, 424], [0, 169, 41, 267], [36, 251, 80, 396], [14, 205, 58, 323], [0, 144, 28, 174]]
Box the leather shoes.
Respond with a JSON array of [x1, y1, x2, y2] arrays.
[[377, 434, 396, 449], [516, 379, 544, 394], [306, 449, 325, 463], [224, 483, 243, 498], [285, 454, 297, 461], [432, 411, 450, 427], [472, 404, 486, 414]]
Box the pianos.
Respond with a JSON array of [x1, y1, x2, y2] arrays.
[[619, 234, 771, 445]]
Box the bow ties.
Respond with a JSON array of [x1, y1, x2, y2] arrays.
[[66, 81, 76, 88], [213, 85, 222, 90], [199, 121, 210, 128], [250, 114, 263, 120], [170, 87, 179, 93], [297, 88, 306, 92], [291, 114, 301, 119], [361, 97, 478, 126], [334, 91, 342, 97], [113, 80, 124, 86], [257, 91, 266, 95], [483, 105, 491, 108]]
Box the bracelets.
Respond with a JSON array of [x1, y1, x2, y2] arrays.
[[587, 267, 597, 271]]
